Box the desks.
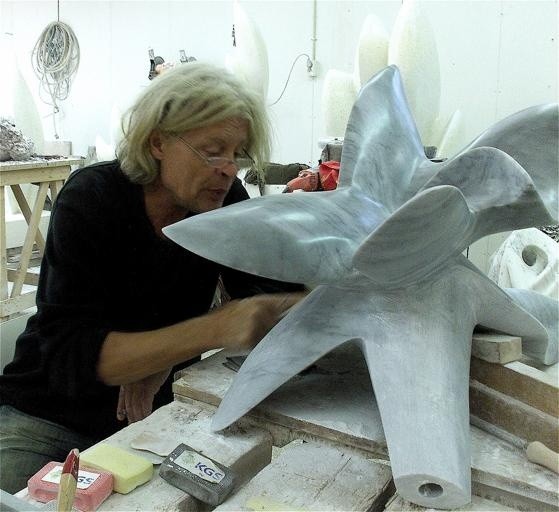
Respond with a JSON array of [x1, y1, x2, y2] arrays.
[[13, 400, 522, 512], [0, 157, 87, 322], [173, 349, 558, 512]]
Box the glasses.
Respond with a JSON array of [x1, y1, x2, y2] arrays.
[[171, 131, 255, 168]]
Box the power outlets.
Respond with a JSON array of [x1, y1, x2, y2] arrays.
[[307, 61, 319, 76]]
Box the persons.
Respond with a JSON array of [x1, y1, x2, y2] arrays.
[[0, 62, 306, 495]]
